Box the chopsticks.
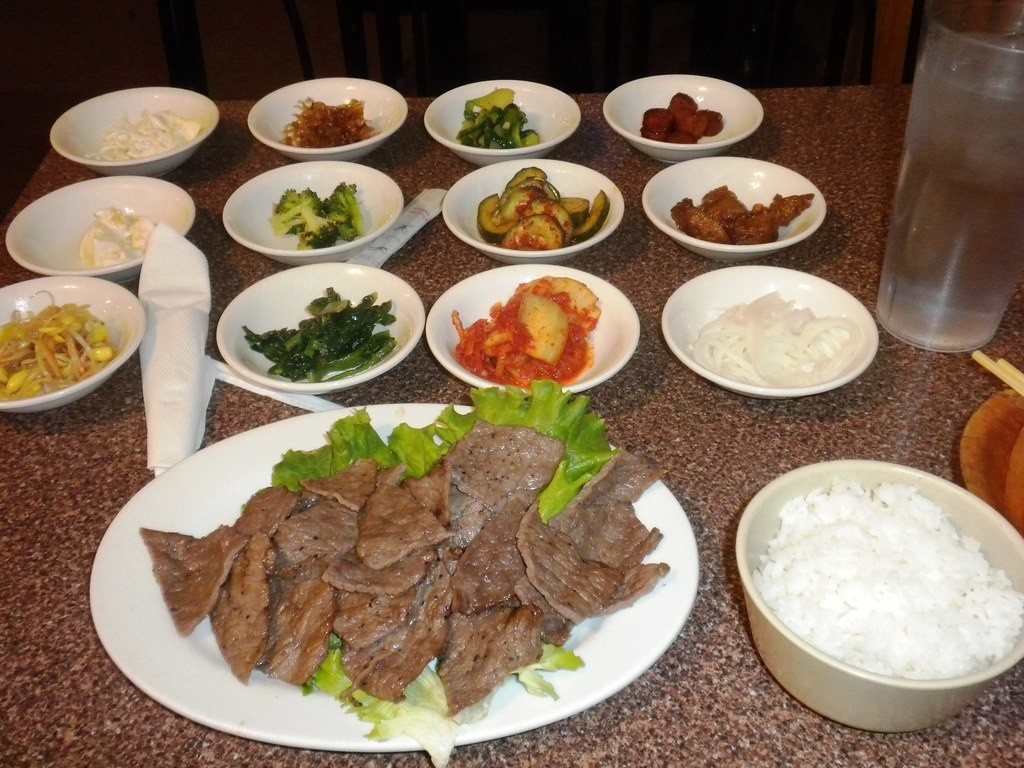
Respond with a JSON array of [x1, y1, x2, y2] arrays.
[[971, 350, 1024, 398]]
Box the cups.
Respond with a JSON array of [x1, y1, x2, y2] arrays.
[[875, 0, 1024, 350]]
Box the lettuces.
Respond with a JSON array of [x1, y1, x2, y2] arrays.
[[245, 381, 625, 768]]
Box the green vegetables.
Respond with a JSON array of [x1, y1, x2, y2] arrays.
[[455, 88, 539, 150], [242, 287, 396, 384]]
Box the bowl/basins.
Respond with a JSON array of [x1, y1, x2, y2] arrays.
[[5, 175, 196, 284], [734, 461, 1024, 731], [424, 79, 581, 165], [641, 157, 827, 259], [661, 265, 878, 398], [603, 74, 764, 163], [223, 160, 403, 266], [0, 275, 146, 415], [442, 160, 625, 264], [217, 262, 425, 392], [247, 76, 409, 161], [426, 265, 640, 393], [50, 85, 219, 177]]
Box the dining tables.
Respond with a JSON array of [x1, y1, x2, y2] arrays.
[[0, 83, 1023, 767]]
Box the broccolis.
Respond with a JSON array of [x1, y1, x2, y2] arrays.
[[270, 182, 366, 249]]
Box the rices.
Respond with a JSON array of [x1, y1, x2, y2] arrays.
[[751, 481, 1024, 678]]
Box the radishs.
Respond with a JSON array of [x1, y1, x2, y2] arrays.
[[692, 292, 860, 389]]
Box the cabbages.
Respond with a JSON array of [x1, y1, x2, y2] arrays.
[[80, 108, 203, 162]]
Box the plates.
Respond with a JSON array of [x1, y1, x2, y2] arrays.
[[960, 388, 1024, 542], [88, 403, 698, 752]]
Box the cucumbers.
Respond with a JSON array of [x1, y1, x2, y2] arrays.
[[478, 167, 609, 249]]
